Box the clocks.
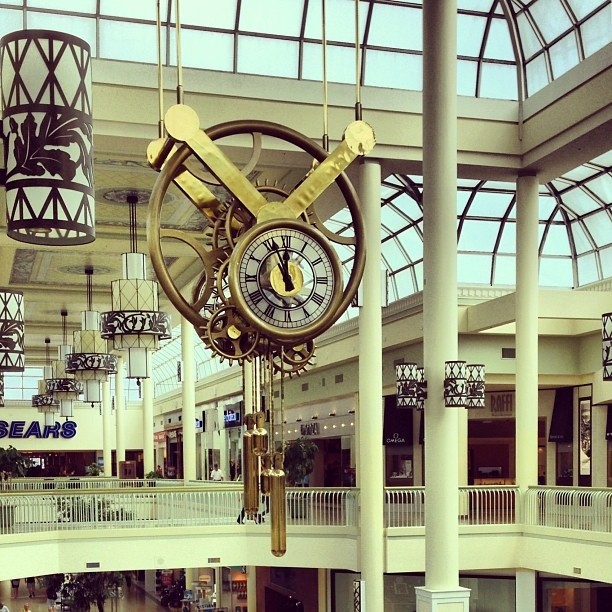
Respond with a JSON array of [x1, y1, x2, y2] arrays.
[[226, 219, 346, 338]]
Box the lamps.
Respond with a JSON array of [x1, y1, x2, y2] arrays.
[[444, 360, 467, 407], [0, 287, 25, 371], [32, 336, 60, 428], [395, 362, 418, 408], [98, 187, 173, 399], [46, 308, 84, 421], [467, 363, 485, 408], [600, 312, 611, 380], [0, 30, 98, 247], [64, 265, 117, 407], [0, 371, 5, 407], [354, 578, 364, 610], [415, 367, 426, 410]]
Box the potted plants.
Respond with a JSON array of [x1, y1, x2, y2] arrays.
[[146, 471, 157, 487], [283, 436, 320, 521]]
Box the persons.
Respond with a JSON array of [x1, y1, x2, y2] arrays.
[[156, 465, 163, 479], [48, 606, 54, 612], [0, 602, 9, 612], [11, 579, 20, 599], [126, 573, 131, 593], [210, 464, 224, 482], [156, 570, 161, 579], [22, 603, 31, 612], [25, 578, 35, 597]]
